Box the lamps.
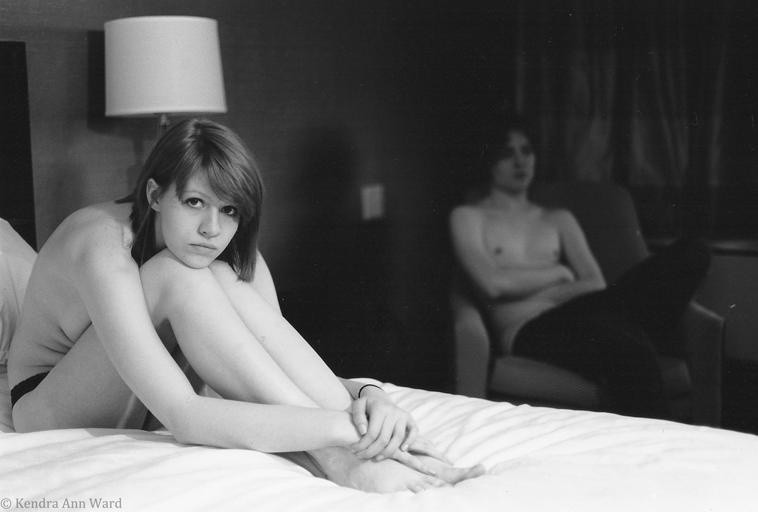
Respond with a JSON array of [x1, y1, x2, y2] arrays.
[[101, 13, 228, 148]]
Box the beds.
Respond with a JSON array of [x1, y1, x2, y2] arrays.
[[1, 37, 758, 500]]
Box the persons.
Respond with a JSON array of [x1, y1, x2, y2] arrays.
[[7, 118, 484, 495], [448, 123, 724, 419]]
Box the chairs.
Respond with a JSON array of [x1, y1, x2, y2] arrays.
[[446, 178, 726, 432]]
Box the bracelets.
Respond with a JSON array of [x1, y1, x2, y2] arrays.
[[358, 384, 384, 399]]
